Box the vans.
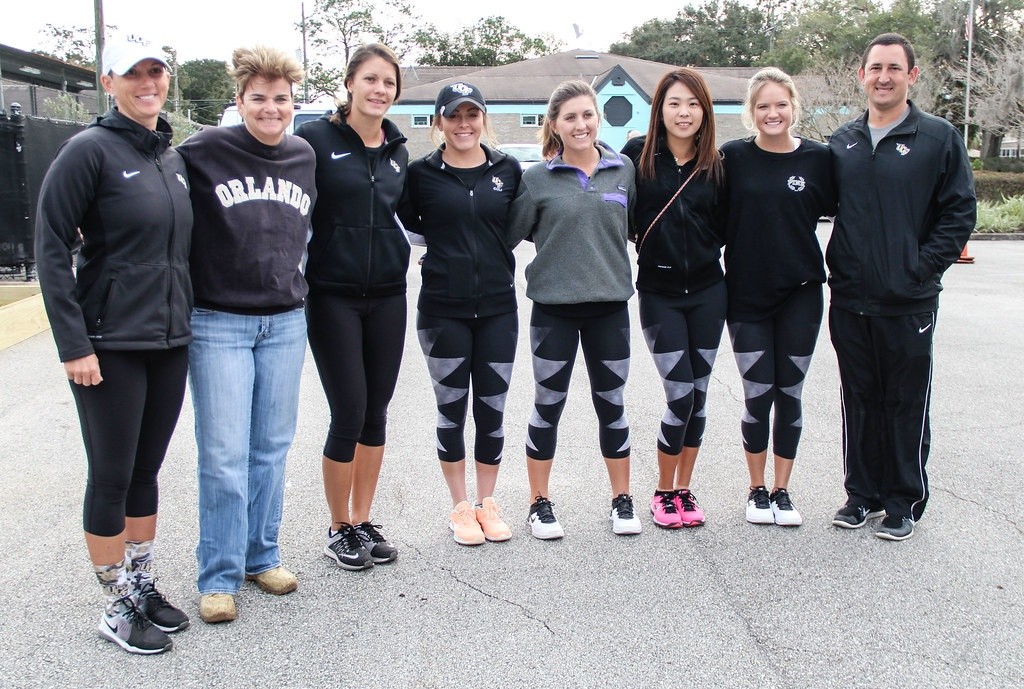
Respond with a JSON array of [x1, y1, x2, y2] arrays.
[[218, 102, 336, 134]]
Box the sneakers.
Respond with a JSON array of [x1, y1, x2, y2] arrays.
[[473, 496, 513, 541], [131, 576, 190, 632], [609, 493, 642, 534], [354, 518, 398, 564], [875, 514, 920, 541], [200, 591, 238, 623], [649, 489, 683, 529], [832, 502, 886, 528], [746, 484, 774, 524], [323, 520, 375, 570], [525, 491, 565, 539], [674, 488, 706, 527], [244, 566, 298, 595], [770, 487, 802, 526], [449, 501, 485, 545], [97, 594, 172, 655]]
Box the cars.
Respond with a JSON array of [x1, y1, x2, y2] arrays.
[[497, 145, 546, 171]]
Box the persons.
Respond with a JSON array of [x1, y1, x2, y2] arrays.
[[718, 66, 833, 526], [77, 45, 317, 620], [398, 84, 534, 546], [509, 78, 641, 540], [293, 43, 408, 570], [619, 70, 727, 530], [826, 34, 977, 540], [35, 33, 189, 653], [628, 129, 640, 141]]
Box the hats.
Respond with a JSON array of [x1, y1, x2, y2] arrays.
[[100, 28, 173, 76], [434, 81, 487, 118]]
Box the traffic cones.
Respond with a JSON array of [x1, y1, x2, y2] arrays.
[[956, 243, 974, 264]]
